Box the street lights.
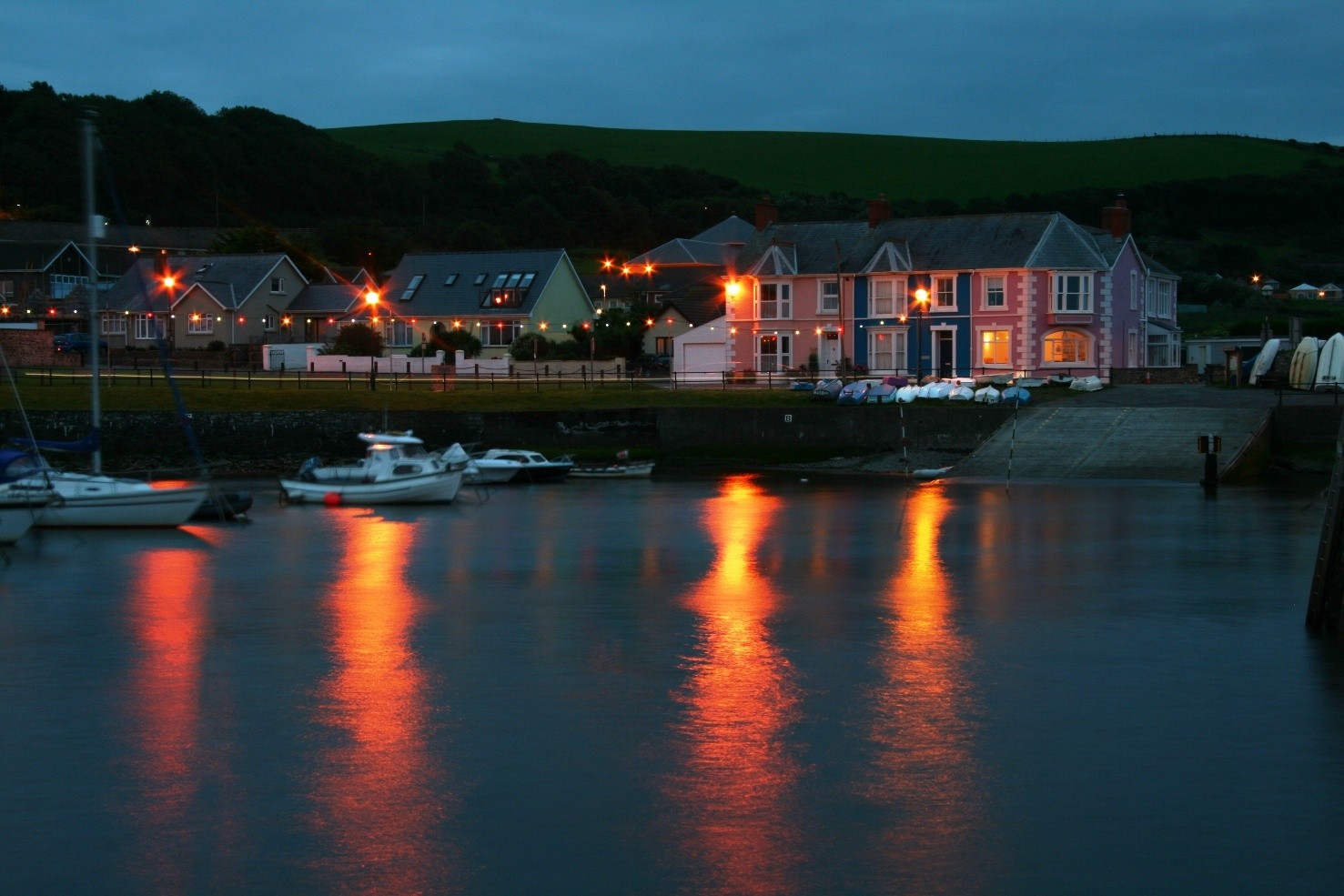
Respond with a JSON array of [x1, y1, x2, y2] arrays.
[[160, 270, 180, 357], [1261, 285, 1274, 347], [914, 286, 929, 386], [365, 289, 380, 378]]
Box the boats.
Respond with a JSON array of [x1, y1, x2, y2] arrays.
[[789, 368, 1104, 411], [456, 445, 574, 489], [566, 456, 655, 480], [276, 426, 480, 513], [2, 354, 67, 563], [11, 104, 209, 531]]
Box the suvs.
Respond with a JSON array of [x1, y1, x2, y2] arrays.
[[53, 332, 109, 354], [646, 355, 674, 380]]
